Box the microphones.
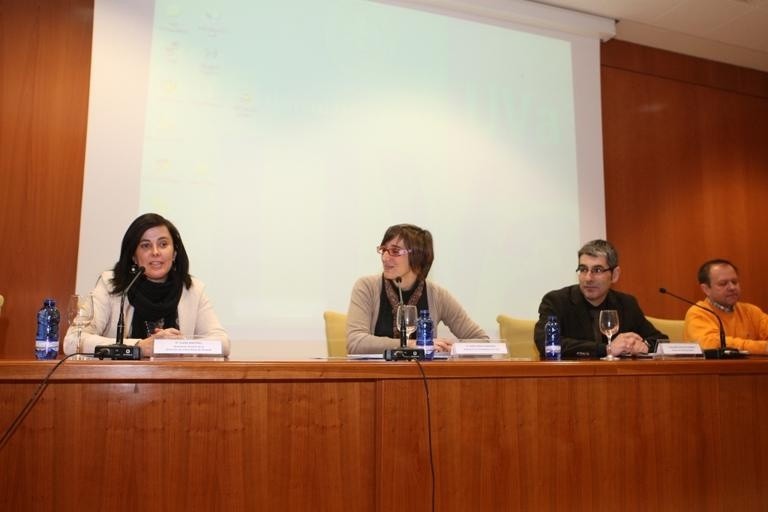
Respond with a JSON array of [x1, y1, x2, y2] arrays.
[[659, 287, 739, 360], [384, 276, 425, 359], [92, 265, 145, 359]]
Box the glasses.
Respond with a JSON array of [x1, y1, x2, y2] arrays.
[[576, 268, 611, 275], [377, 246, 412, 257]]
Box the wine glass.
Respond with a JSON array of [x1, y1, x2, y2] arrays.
[[598, 309, 620, 361], [396, 305, 419, 348], [66, 295, 94, 360]]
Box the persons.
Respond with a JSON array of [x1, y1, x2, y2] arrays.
[[534, 239, 670, 360], [683, 259, 768, 355], [344, 224, 491, 356], [63, 213, 230, 360]]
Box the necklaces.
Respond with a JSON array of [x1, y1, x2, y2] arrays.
[[145, 318, 165, 337]]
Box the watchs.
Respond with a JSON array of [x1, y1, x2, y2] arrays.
[[643, 339, 653, 353]]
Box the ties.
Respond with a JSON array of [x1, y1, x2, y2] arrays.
[[590, 310, 603, 344]]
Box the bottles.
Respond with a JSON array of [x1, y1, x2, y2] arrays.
[[35, 298, 60, 361], [415, 310, 434, 362], [544, 316, 562, 360]]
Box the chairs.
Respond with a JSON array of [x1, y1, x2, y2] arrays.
[[324, 310, 349, 359], [497, 314, 542, 360], [644, 316, 688, 344]]
